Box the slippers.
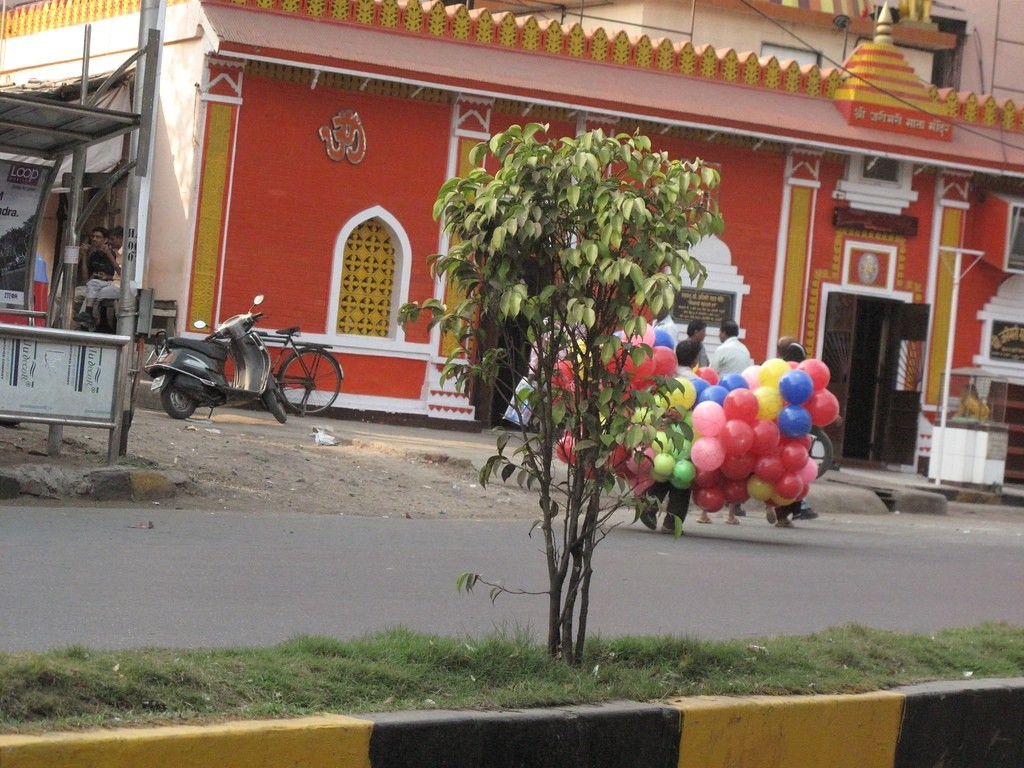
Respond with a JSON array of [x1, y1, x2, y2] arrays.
[[724, 518, 740, 524], [696, 517, 711, 523]]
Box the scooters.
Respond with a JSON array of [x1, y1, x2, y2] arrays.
[[143, 294, 288, 424]]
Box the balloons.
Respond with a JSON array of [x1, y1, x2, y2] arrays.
[[550, 323, 838, 513]]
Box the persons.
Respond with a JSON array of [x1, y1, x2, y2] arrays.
[[640, 339, 701, 536], [682, 318, 751, 523], [73, 226, 123, 332], [766, 336, 818, 529]]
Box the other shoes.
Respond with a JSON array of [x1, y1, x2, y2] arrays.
[[801, 508, 817, 520], [636, 505, 658, 530], [72, 312, 95, 324], [734, 508, 746, 516], [662, 519, 684, 533], [778, 521, 793, 527], [765, 504, 775, 523], [89, 324, 112, 332]]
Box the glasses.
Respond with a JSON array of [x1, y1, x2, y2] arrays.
[[90, 234, 105, 239]]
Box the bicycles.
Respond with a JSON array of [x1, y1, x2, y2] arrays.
[[261, 325, 344, 417]]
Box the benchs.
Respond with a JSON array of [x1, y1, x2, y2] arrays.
[[74, 297, 176, 340]]
